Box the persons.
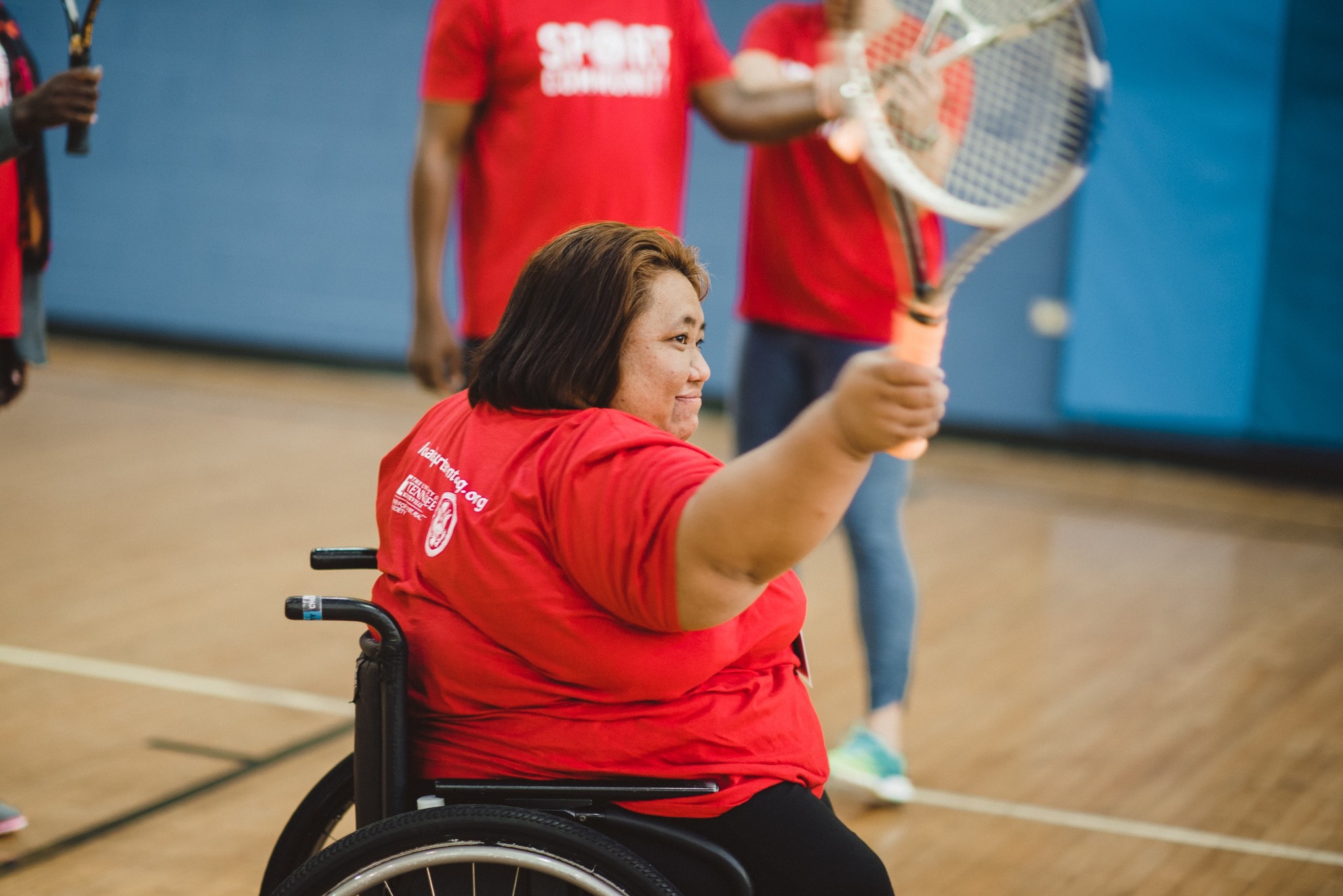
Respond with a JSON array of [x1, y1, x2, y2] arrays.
[[367, 221, 949, 895], [736, 0, 971, 809], [406, 0, 854, 401], [0, 0, 102, 408]]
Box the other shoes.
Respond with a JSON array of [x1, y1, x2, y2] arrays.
[[826, 724, 913, 803]]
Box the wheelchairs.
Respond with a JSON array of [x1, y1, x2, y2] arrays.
[[258, 546, 752, 896]]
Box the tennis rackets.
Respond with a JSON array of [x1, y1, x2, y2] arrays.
[[827, 0, 1081, 166], [63, 0, 103, 157], [842, 0, 1112, 461]]
